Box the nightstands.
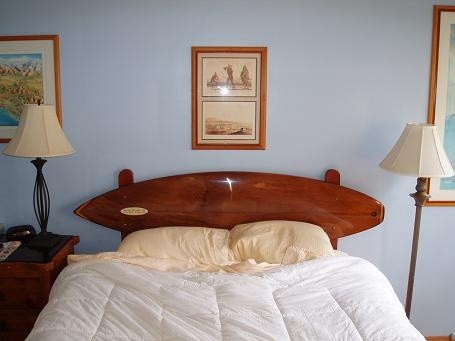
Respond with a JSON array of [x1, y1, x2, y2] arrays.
[[0, 235, 80, 340]]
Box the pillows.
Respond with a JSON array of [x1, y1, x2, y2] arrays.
[[231, 220, 333, 264], [117, 226, 235, 265]]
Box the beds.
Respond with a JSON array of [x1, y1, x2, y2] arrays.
[[25, 167, 427, 340]]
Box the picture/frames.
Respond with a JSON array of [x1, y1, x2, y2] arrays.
[[0, 35, 62, 143], [422, 5, 455, 207], [191, 46, 267, 149]]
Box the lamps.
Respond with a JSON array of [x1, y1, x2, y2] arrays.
[[0, 99, 76, 250], [380, 123, 455, 319]]
